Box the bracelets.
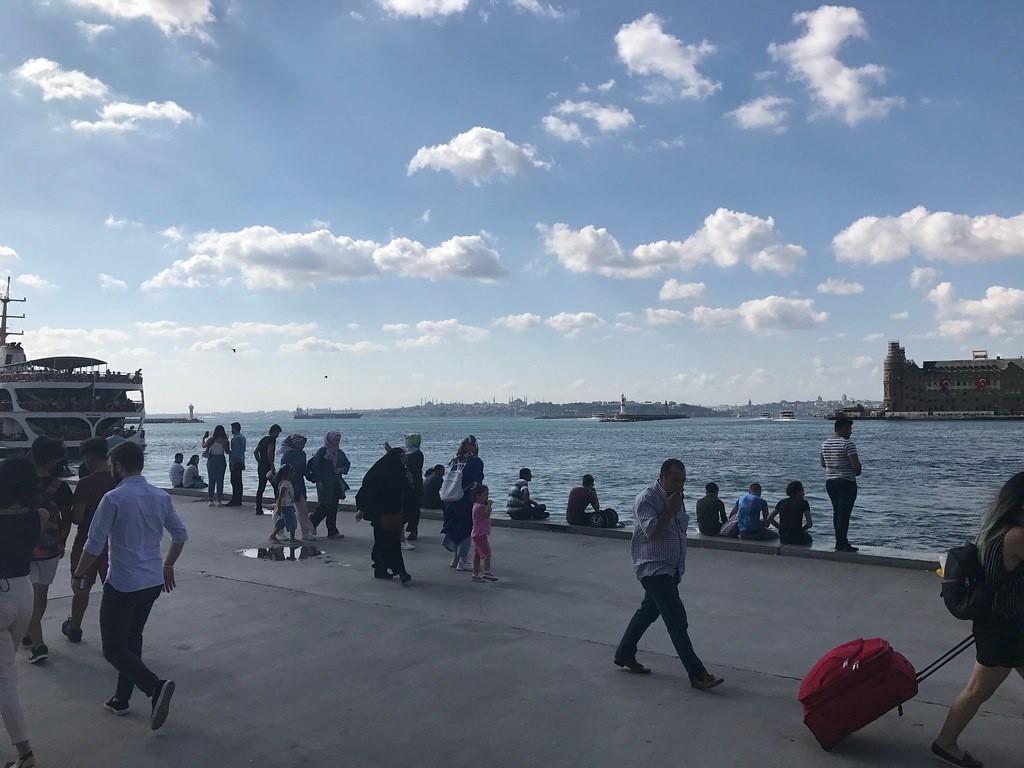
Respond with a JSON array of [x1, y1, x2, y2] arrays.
[[163, 564, 174, 568], [73, 574, 82, 579]]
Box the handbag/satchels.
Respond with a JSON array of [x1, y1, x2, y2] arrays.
[[720, 515, 738, 536], [192, 476, 203, 488], [590, 508, 624, 528], [202, 438, 212, 458]]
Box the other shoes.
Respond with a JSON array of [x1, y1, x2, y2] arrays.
[[209, 500, 223, 507], [255, 509, 263, 514], [400, 542, 415, 550], [225, 501, 242, 507], [449, 559, 499, 582], [614, 657, 650, 673], [930, 741, 983, 768], [399, 572, 411, 582], [837, 547, 858, 552], [267, 530, 345, 544], [690, 673, 723, 688], [373, 570, 392, 578], [407, 534, 417, 540]]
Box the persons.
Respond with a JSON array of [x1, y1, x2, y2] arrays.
[[819, 418, 862, 553], [502, 468, 551, 520], [566, 474, 601, 526], [730, 483, 779, 539], [613, 459, 724, 690], [0, 363, 498, 768], [767, 481, 814, 543], [695, 482, 727, 537], [931, 471, 1024, 768]]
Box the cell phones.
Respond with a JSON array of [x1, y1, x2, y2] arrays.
[[206, 431, 209, 437], [591, 487, 595, 492]]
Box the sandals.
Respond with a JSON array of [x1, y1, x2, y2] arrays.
[[4, 751, 33, 768]]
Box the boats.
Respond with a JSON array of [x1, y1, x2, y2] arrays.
[[778, 410, 796, 421], [758, 412, 771, 419], [0, 277, 147, 458], [293, 404, 363, 418]]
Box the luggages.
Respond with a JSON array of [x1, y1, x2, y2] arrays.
[[798, 635, 977, 752]]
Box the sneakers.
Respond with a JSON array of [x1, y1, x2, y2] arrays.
[[61, 618, 82, 641], [29, 646, 48, 664], [21, 636, 33, 648], [104, 695, 130, 714], [150, 680, 176, 729]]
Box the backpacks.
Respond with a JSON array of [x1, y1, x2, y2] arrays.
[[940, 535, 1021, 621], [440, 461, 474, 501]]
